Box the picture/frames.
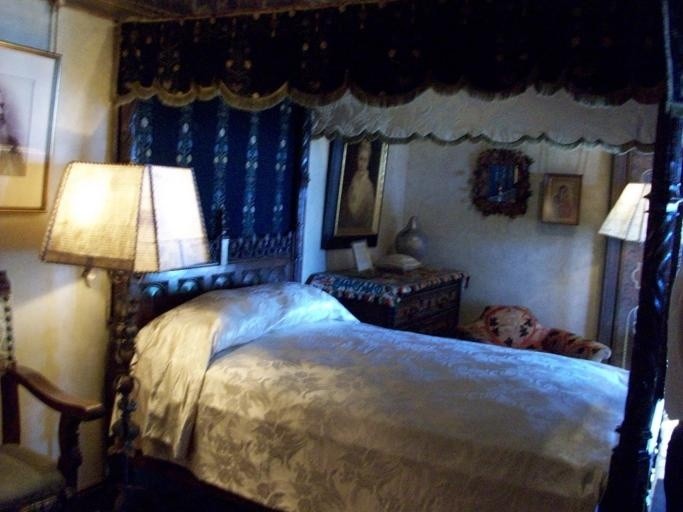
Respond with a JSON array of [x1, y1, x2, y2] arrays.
[[0, 39, 63, 213], [541, 173, 583, 225], [467, 148, 534, 219], [322, 138, 389, 250]]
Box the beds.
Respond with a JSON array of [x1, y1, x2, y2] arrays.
[[108, 254, 663, 512]]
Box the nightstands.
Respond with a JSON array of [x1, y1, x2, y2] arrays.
[[308, 267, 470, 338]]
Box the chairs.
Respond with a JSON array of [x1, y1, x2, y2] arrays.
[[0, 270, 106, 512], [455, 305, 611, 363]]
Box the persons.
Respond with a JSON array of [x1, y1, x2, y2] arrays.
[[347, 140, 373, 233]]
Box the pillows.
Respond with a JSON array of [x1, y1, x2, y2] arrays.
[[110, 280, 359, 460]]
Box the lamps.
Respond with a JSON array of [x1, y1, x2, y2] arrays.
[[39, 160, 212, 512], [598, 182, 651, 244]]
